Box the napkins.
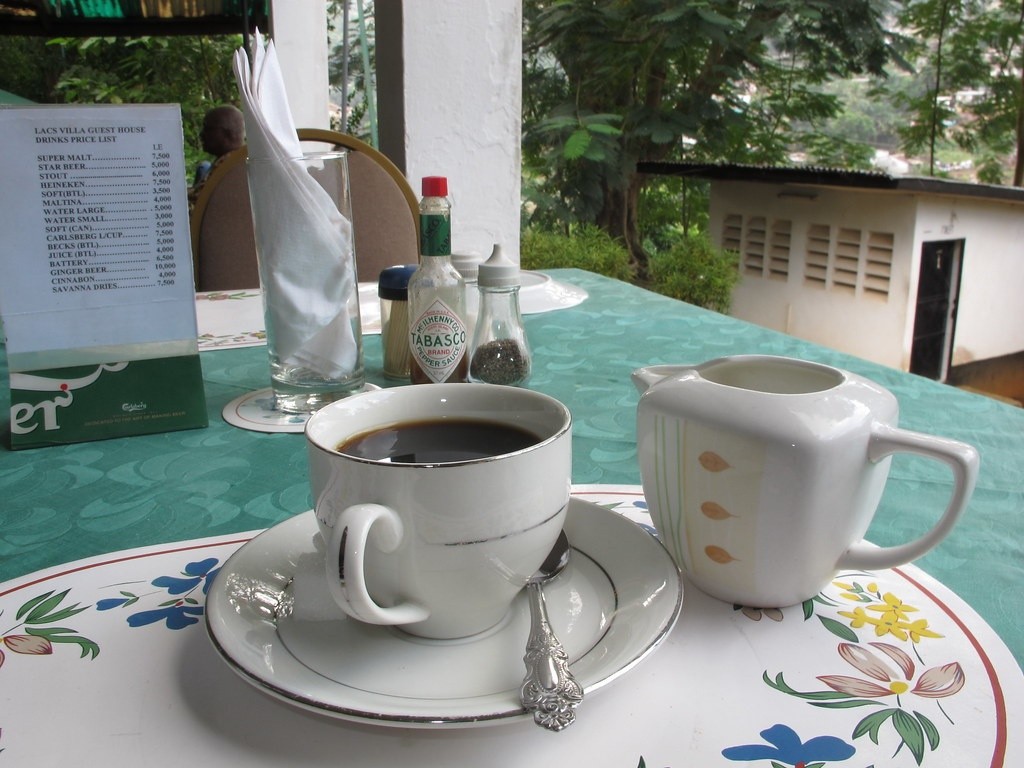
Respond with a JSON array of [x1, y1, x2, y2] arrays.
[[231, 25, 357, 384]]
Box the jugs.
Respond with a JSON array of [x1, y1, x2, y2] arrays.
[[628, 353, 979, 608]]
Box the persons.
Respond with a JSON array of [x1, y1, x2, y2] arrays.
[[198, 105, 246, 158]]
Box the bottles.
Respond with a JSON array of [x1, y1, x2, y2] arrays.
[[406, 176, 469, 385], [247, 152, 364, 416], [377, 263, 419, 382], [466, 243, 533, 390]]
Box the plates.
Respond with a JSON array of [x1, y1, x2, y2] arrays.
[[202, 496, 685, 729]]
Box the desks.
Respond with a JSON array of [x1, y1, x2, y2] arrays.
[[0, 266, 1024, 768]]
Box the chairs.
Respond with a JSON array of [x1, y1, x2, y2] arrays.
[[191, 128, 420, 291]]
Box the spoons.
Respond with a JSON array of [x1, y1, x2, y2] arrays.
[[518, 527, 585, 732]]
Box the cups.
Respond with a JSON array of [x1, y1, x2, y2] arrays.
[[303, 382, 572, 640]]
